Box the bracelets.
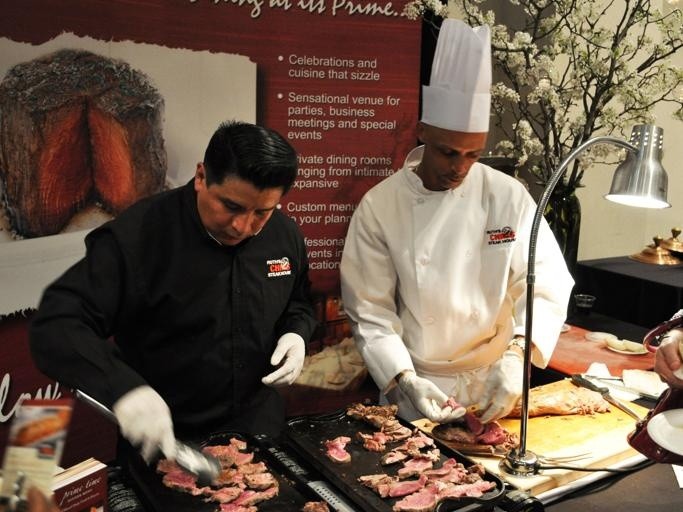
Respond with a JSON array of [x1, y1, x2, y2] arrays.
[[507, 338, 525, 353]]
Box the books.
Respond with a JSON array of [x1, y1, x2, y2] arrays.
[[40, 455, 108, 512]]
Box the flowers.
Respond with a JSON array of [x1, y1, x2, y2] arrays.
[[401, 0, 682, 184]]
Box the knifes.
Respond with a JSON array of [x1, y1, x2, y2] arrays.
[[571, 373, 641, 422]]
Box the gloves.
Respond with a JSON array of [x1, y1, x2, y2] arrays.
[[478, 350, 524, 425], [261, 332, 306, 387], [114, 386, 177, 465], [398, 372, 467, 423]]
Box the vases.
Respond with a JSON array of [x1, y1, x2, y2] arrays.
[[542, 184, 583, 314]]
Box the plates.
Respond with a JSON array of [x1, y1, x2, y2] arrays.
[[583, 331, 618, 343], [605, 340, 648, 357]]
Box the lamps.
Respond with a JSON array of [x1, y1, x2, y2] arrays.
[[499, 124, 673, 476]]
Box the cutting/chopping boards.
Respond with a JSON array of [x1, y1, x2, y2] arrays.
[[409, 376, 651, 497]]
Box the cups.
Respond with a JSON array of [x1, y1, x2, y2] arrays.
[[574, 294, 595, 316]]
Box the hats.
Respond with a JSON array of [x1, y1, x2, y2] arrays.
[[419, 18, 493, 134]]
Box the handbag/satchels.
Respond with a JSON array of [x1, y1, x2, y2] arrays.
[[626, 317, 683, 466]]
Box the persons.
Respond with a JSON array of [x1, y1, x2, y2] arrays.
[[338, 119, 577, 434], [651, 308, 683, 393], [27, 118, 320, 470]]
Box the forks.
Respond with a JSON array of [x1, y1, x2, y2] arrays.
[[438, 432, 591, 467]]
